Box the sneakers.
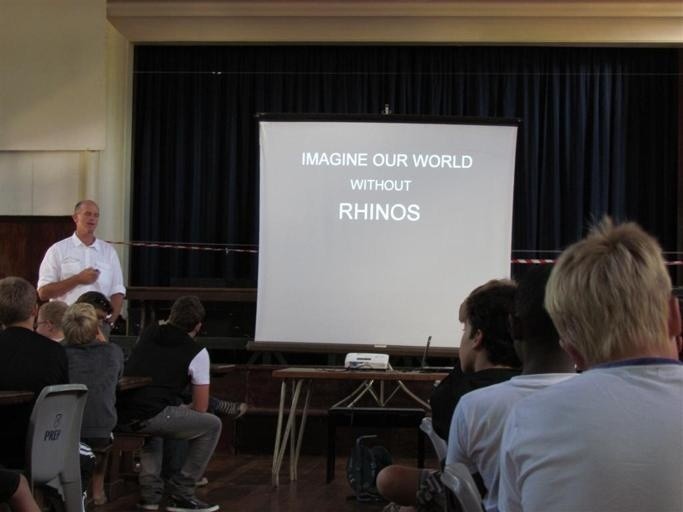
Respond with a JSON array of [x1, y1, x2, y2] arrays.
[[211, 397, 249, 420], [135, 498, 160, 511], [164, 491, 220, 512]]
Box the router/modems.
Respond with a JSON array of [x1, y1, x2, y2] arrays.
[[420, 335, 457, 373]]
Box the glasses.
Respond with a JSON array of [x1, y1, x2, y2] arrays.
[[32, 321, 46, 329]]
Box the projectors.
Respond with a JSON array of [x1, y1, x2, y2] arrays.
[[344, 351, 391, 371]]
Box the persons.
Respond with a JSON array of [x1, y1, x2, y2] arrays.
[[444, 261, 578, 512], [36, 200, 127, 342], [496, 210, 683, 512], [374, 276, 523, 512]]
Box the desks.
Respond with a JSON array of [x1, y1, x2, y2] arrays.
[[268, 366, 455, 491], [0, 362, 239, 510]]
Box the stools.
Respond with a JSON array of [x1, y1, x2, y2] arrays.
[[324, 404, 427, 486]]
